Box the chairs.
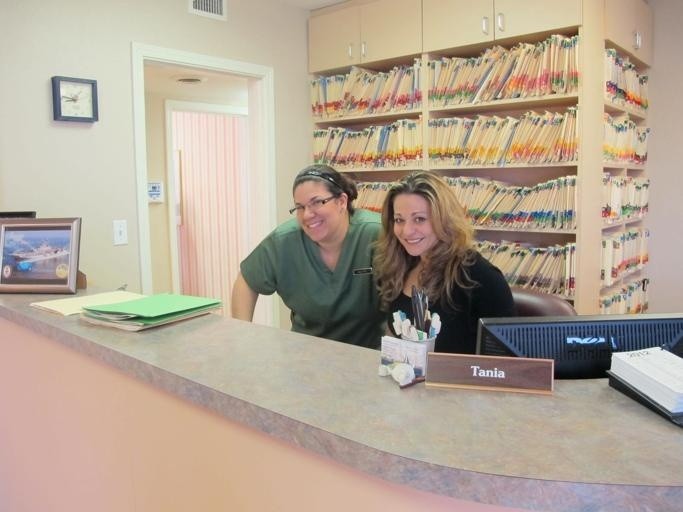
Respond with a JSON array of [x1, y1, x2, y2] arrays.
[[510, 287, 575, 315]]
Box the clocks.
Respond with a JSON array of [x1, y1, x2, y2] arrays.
[[52, 76, 98, 122]]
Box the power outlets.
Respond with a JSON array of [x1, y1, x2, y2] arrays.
[[115, 218, 128, 246]]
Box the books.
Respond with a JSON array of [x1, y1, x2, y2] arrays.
[[308, 32, 577, 314], [77, 292, 223, 333], [604, 47, 652, 314]]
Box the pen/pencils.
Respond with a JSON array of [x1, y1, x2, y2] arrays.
[[392, 284, 441, 339]]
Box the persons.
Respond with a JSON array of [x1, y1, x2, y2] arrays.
[[358, 170, 517, 354], [230, 163, 384, 350]]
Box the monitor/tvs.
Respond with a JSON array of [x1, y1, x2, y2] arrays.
[[474, 311, 683, 382]]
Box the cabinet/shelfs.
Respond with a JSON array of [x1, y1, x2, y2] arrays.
[[309, 0, 654, 315]]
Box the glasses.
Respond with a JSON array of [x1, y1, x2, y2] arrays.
[[289, 194, 337, 215]]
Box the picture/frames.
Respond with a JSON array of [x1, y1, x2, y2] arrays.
[[0, 217, 81, 293]]
